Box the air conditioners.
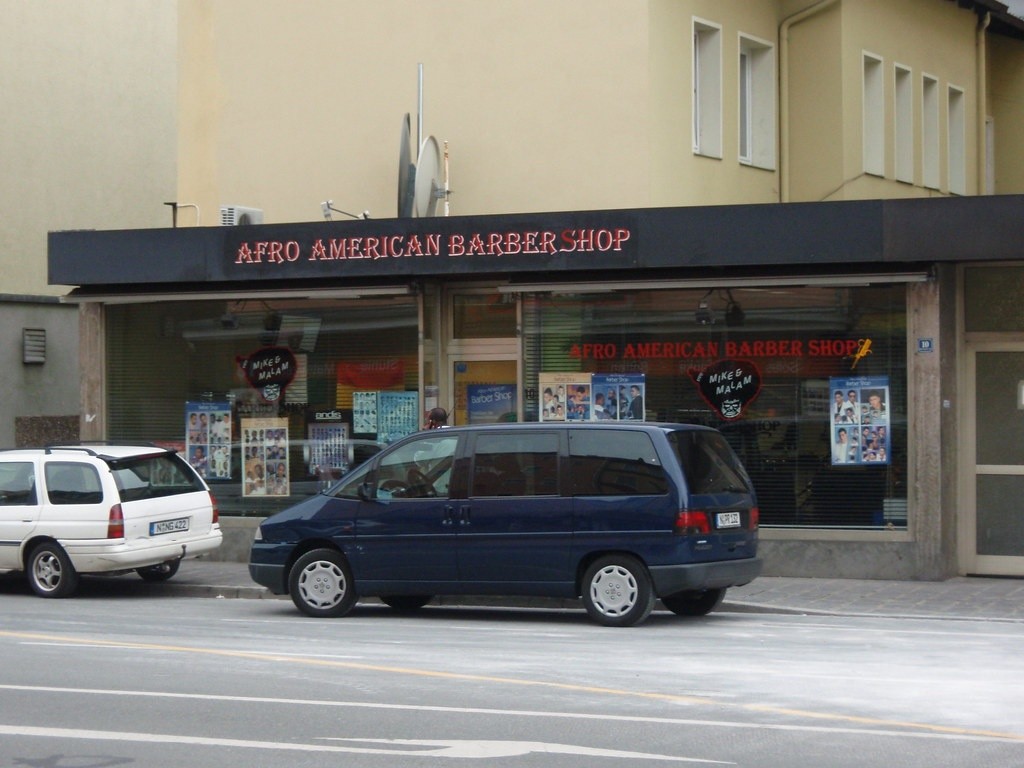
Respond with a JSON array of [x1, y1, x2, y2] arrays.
[[219, 206, 263, 226]]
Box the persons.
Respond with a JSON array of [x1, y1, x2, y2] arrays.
[[541, 384, 643, 422], [413, 408, 458, 495], [245, 429, 287, 495], [189, 413, 231, 477], [834, 389, 886, 462]]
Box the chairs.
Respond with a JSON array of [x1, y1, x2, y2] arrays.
[[473, 452, 526, 496]]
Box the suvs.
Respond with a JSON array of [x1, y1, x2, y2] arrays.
[[0, 440, 223, 599], [171, 439, 406, 495]]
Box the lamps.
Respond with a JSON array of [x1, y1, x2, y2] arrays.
[[219, 299, 243, 328], [260, 301, 284, 332], [694, 287, 717, 325], [725, 289, 746, 327]]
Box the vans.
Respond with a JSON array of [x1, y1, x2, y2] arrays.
[[715, 417, 884, 528], [247, 420, 766, 628]]
